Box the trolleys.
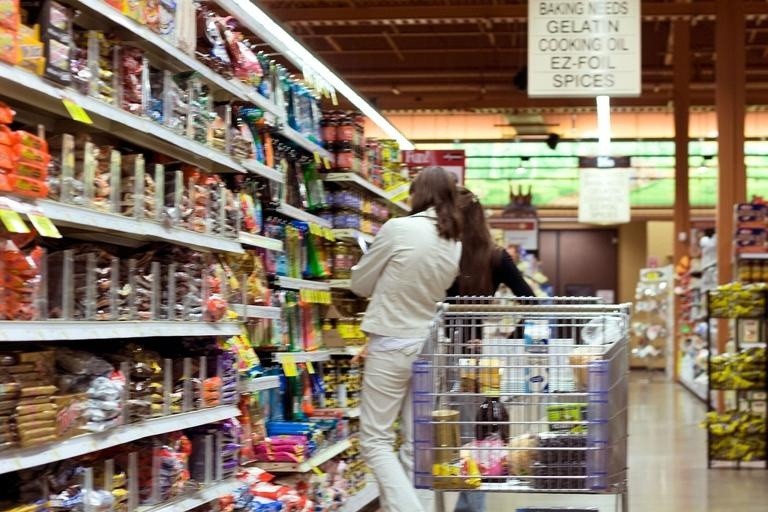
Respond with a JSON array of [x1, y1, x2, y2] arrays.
[[411, 289, 635, 512]]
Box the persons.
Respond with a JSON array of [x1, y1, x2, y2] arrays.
[[505, 243, 520, 267], [519, 249, 558, 341], [438, 186, 541, 512], [346, 166, 469, 512]]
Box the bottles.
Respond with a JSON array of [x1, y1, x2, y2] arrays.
[[476, 395, 510, 483]]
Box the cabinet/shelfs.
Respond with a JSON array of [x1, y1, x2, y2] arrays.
[[674, 204, 768, 469]]
